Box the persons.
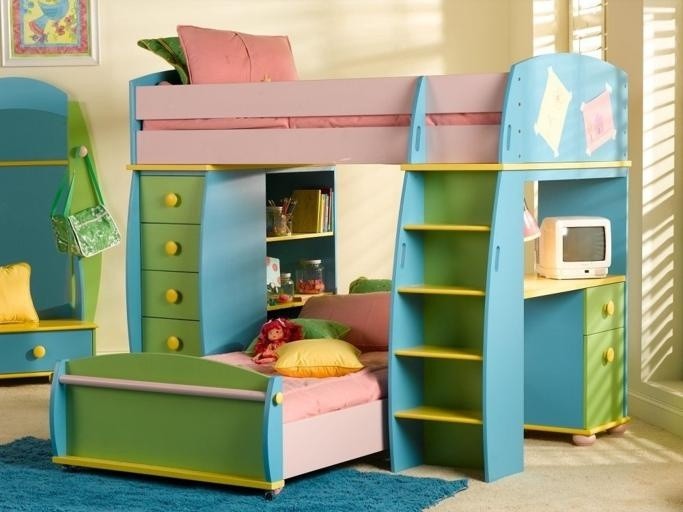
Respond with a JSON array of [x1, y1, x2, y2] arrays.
[[253, 318, 303, 364]]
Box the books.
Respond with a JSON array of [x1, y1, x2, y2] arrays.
[[293, 188, 333, 233]]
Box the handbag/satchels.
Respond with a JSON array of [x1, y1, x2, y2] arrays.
[[47, 204, 121, 258]]
